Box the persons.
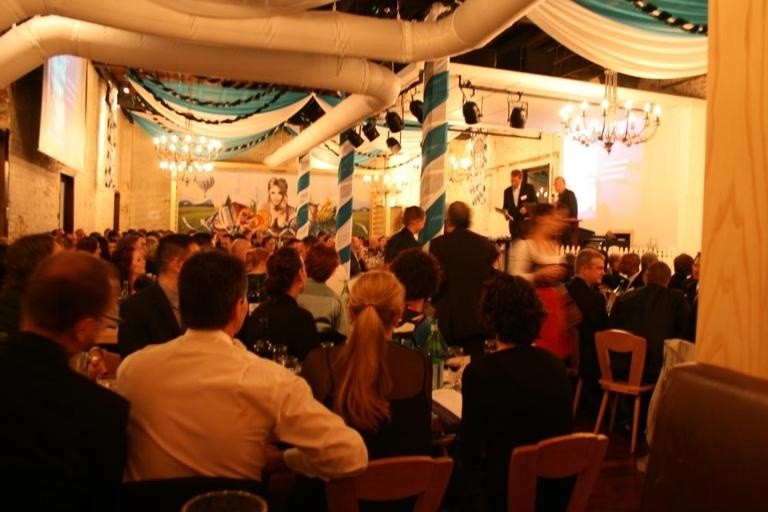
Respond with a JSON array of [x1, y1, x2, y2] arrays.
[[552, 177, 578, 218], [266, 177, 296, 235], [500, 170, 538, 240]]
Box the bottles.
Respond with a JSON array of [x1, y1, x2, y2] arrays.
[[610, 278, 623, 310], [424, 319, 446, 393]]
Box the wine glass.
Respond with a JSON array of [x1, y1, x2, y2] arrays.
[[444, 345, 464, 391]]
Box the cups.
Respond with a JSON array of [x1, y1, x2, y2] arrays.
[[485, 339, 499, 354], [249, 339, 301, 375]]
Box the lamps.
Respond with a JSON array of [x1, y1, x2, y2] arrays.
[[344, 78, 423, 154], [459, 84, 531, 130], [150, 77, 223, 185], [363, 173, 406, 203], [558, 65, 661, 154]]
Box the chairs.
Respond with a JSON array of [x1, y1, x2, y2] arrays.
[[113, 329, 657, 512]]
